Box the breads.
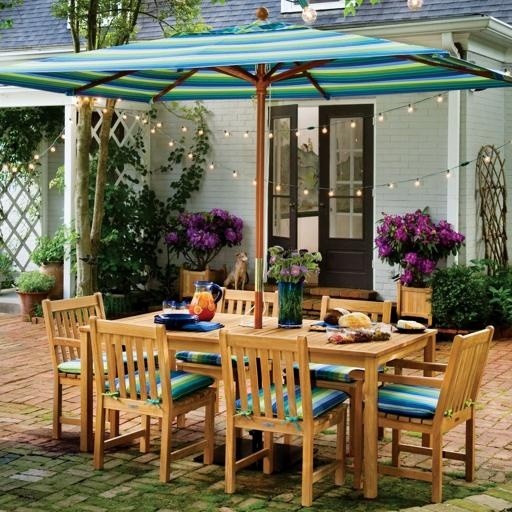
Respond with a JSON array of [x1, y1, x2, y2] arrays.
[[339, 312, 372, 327], [324, 307, 352, 325]]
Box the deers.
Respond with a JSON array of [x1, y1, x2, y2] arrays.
[[223, 241, 248, 290]]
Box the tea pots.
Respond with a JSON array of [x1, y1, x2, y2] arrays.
[[188, 281, 223, 322]]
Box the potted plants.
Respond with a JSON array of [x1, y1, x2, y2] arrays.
[[14, 221, 81, 323]]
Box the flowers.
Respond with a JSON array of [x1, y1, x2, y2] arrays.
[[371, 205, 467, 288], [166, 204, 244, 268], [263, 244, 322, 323]]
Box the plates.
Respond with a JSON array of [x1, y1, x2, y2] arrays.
[[395, 325, 425, 334], [153, 313, 200, 331]]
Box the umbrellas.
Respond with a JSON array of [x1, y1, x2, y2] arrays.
[[0, 6, 512, 329]]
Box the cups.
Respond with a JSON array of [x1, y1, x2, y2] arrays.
[[162, 300, 187, 314]]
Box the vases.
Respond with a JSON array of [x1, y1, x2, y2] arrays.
[[178, 264, 226, 302], [396, 281, 434, 326], [276, 281, 304, 325]]
